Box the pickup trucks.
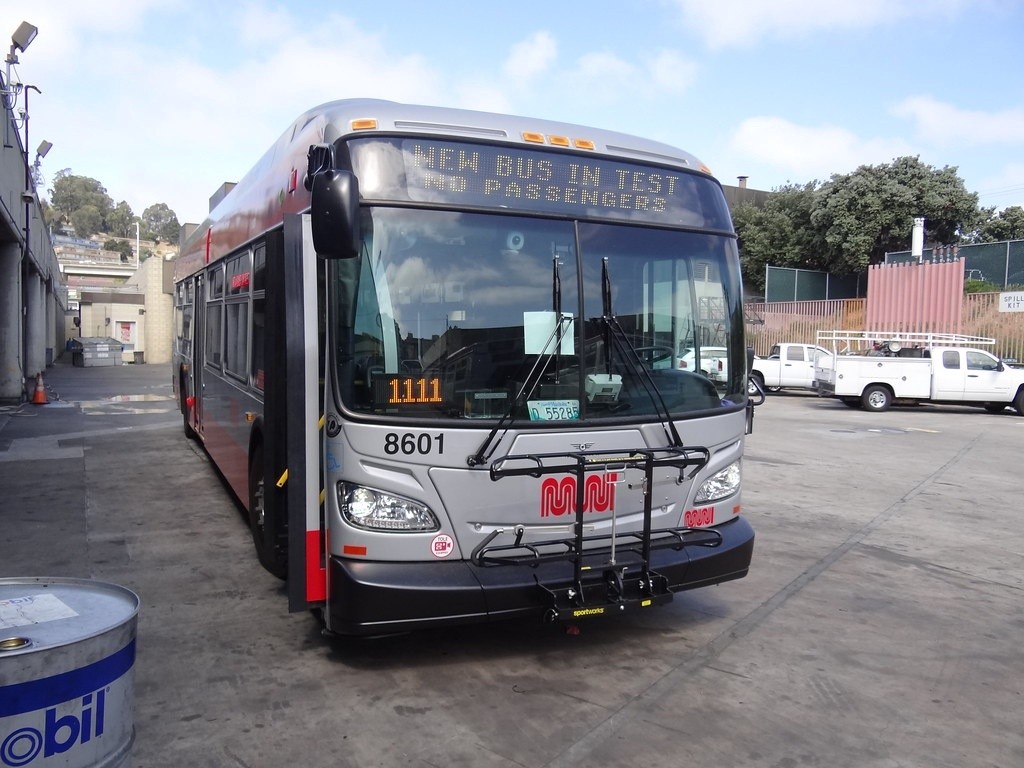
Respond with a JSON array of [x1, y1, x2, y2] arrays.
[[709, 342, 833, 395], [815, 330, 1024, 415]]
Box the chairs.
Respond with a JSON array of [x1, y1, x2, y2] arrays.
[[392, 281, 470, 336]]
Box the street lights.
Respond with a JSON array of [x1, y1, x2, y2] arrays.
[[132, 222, 140, 268]]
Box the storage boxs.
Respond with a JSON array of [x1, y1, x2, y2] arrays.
[[72, 336, 123, 368]]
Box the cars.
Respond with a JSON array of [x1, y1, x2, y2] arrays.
[[677, 346, 761, 383]]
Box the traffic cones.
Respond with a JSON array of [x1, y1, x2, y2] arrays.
[[32, 372, 52, 405]]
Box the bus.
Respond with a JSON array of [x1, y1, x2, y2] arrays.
[[171, 99, 767, 643]]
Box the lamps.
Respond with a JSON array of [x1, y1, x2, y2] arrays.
[[32, 139, 53, 220], [21, 190, 35, 204], [4, 21, 38, 148]]
